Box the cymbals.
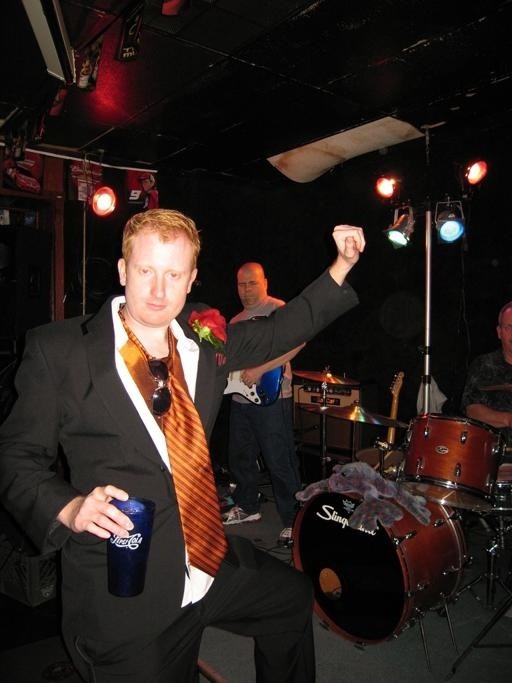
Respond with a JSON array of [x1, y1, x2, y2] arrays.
[[479, 385, 512, 389], [296, 402, 404, 427], [292, 369, 359, 386]]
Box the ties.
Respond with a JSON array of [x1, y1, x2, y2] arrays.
[[117, 309, 227, 576]]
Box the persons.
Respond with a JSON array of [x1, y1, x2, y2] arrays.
[[139, 173, 159, 210], [0, 210, 365, 683], [219, 262, 306, 549], [463, 303, 512, 524]]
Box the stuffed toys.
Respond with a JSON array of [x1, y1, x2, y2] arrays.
[[295, 463, 431, 532]]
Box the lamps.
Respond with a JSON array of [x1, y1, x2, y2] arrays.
[[375, 169, 405, 202], [453, 151, 489, 190], [381, 214, 413, 248], [85, 185, 118, 219], [435, 210, 466, 241]]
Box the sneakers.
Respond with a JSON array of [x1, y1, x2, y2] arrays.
[[277, 527, 293, 547], [222, 506, 261, 525]]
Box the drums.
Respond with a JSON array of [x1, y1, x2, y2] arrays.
[[352, 449, 403, 479], [286, 487, 468, 650], [407, 414, 502, 499]]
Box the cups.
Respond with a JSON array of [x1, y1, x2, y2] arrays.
[[107, 496, 156, 596]]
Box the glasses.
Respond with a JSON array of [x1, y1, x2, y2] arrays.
[[149, 360, 170, 414]]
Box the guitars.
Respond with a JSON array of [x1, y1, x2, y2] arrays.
[[356, 370, 404, 442], [224, 362, 283, 406]]
